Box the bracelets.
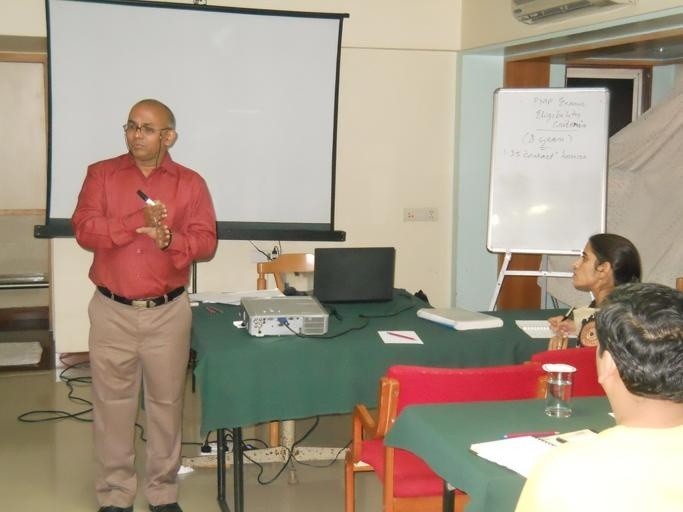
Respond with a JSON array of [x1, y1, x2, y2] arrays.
[[161, 223, 173, 251]]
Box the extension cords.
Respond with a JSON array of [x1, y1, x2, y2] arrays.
[[271, 248, 279, 259], [201, 442, 233, 455]]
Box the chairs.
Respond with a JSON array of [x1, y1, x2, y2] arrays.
[[676, 277, 683, 291], [256, 252, 314, 448]]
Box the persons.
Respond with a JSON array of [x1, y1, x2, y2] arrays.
[[70, 98, 218, 512], [548, 233, 643, 355], [512, 280, 683, 512]]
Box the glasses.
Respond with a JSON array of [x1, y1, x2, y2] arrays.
[[123, 123, 171, 136]]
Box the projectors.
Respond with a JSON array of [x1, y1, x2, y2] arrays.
[[239, 295, 329, 338]]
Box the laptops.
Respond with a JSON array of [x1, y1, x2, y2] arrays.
[[315, 248, 395, 302]]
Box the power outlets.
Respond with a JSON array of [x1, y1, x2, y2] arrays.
[[270, 251, 279, 260], [404, 206, 436, 222]]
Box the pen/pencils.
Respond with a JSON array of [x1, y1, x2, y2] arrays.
[[503, 432, 561, 439], [562, 306, 575, 321], [388, 331, 417, 341]]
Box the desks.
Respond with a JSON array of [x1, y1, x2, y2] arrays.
[[191, 288, 683, 512]]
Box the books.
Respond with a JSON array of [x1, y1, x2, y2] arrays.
[[471, 427, 604, 481], [377, 327, 423, 346], [416, 302, 504, 333], [514, 317, 580, 340]]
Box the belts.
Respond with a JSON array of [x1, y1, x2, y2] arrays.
[[98, 286, 185, 308]]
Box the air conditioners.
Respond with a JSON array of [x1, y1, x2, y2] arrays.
[[511, 0, 638, 26]]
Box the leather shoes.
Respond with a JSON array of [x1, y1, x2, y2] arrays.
[[98, 506, 133, 511], [149, 503, 183, 512]]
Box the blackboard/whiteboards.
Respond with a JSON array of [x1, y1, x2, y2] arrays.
[[486, 86, 610, 256]]
